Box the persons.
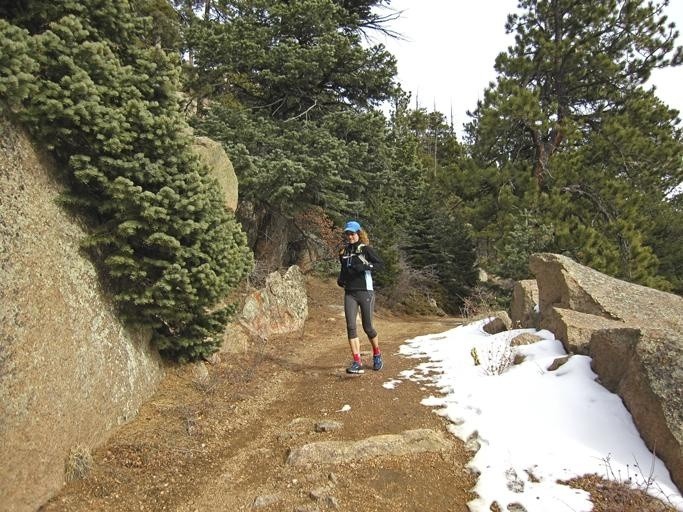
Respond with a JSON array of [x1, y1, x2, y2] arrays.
[[337, 220, 385, 374]]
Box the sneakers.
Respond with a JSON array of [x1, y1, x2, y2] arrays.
[[373, 350, 383, 371], [347, 362, 364, 374]]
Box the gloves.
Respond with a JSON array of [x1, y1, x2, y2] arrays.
[[337, 279, 344, 287], [353, 264, 370, 273]]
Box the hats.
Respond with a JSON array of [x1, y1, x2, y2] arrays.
[[344, 221, 361, 234]]
